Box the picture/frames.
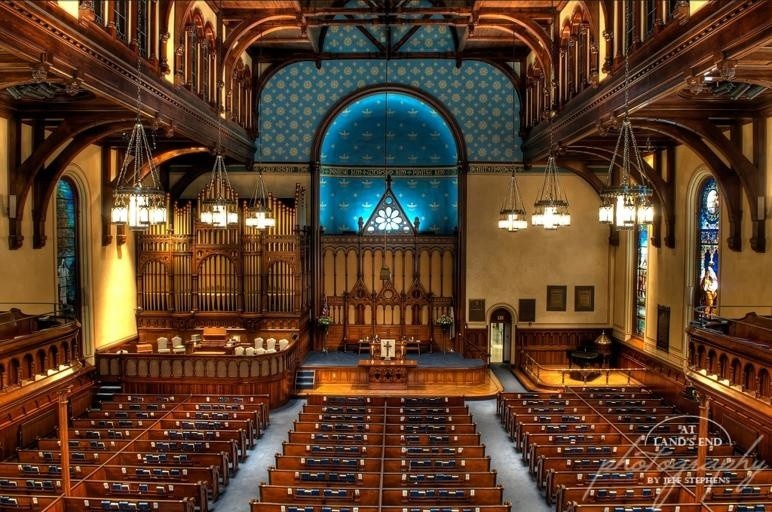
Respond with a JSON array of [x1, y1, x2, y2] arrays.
[[469, 298, 485, 322], [574, 285, 594, 312], [546, 285, 566, 312], [519, 298, 536, 322], [656, 303, 670, 352]]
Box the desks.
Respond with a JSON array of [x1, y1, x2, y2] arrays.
[[357, 361, 418, 390], [568, 349, 603, 376], [357, 340, 421, 356]]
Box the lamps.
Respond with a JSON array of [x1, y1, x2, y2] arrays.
[[110, 1, 166, 229], [598, 1, 658, 230], [531, 0, 572, 229], [245, 25, 276, 229], [200, 1, 239, 228], [380, 93, 389, 280], [497, 21, 528, 227]]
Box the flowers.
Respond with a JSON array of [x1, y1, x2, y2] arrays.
[[437, 315, 453, 324], [318, 317, 332, 325]]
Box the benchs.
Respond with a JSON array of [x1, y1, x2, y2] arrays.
[[1, 391, 269, 512], [249, 392, 512, 512], [495, 388, 772, 511]]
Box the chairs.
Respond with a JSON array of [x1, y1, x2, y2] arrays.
[[136, 336, 289, 356]]
